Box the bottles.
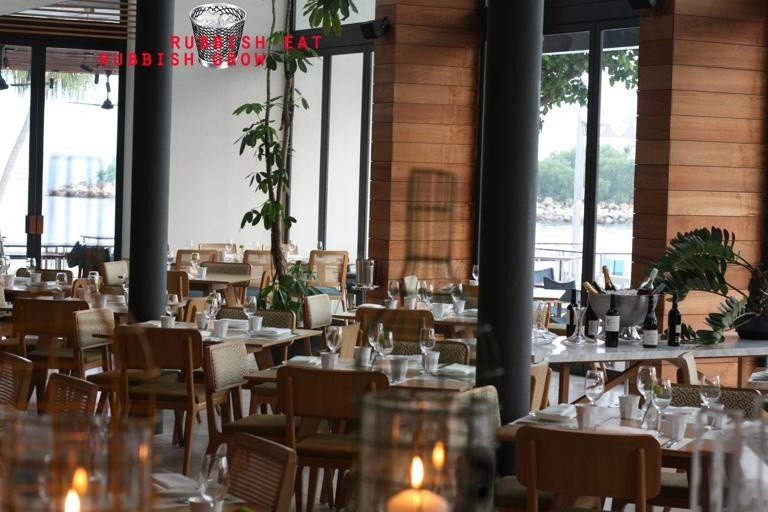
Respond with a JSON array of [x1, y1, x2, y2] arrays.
[[650, 282, 667, 295], [584, 303, 599, 338], [602, 265, 616, 291], [604, 294, 620, 347], [582, 281, 599, 295], [668, 293, 681, 346], [643, 295, 659, 348], [567, 289, 579, 337], [637, 267, 660, 294], [591, 281, 606, 294]]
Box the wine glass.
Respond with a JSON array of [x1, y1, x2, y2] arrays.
[[387, 279, 400, 299], [197, 453, 231, 512], [699, 375, 721, 408], [418, 327, 436, 353], [185, 239, 193, 249], [89, 271, 98, 280], [164, 294, 178, 316], [326, 325, 343, 353], [427, 284, 434, 300], [376, 331, 394, 358], [472, 265, 479, 285], [0, 258, 5, 277], [289, 239, 296, 249], [451, 280, 463, 300], [3, 256, 10, 275], [242, 296, 258, 329], [584, 370, 605, 405], [367, 322, 383, 350], [87, 275, 96, 279], [191, 252, 200, 273], [651, 379, 672, 432], [203, 298, 217, 325], [121, 272, 128, 305], [25, 257, 35, 277], [415, 279, 429, 301], [55, 272, 67, 299], [224, 239, 233, 254], [634, 365, 657, 403], [209, 293, 221, 316]]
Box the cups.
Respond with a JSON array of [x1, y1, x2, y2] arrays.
[[211, 320, 228, 338], [353, 346, 372, 367], [403, 297, 417, 309], [31, 273, 40, 283], [94, 294, 109, 308], [1, 420, 153, 511], [2, 276, 14, 289], [618, 394, 641, 419], [359, 391, 497, 512], [383, 299, 398, 310], [159, 315, 176, 328], [663, 411, 688, 440], [389, 358, 409, 381], [197, 266, 207, 277], [452, 300, 466, 314], [320, 352, 339, 369], [187, 496, 210, 512], [195, 311, 210, 330], [432, 303, 443, 319], [575, 404, 598, 430], [424, 351, 441, 371], [250, 315, 263, 331]]
